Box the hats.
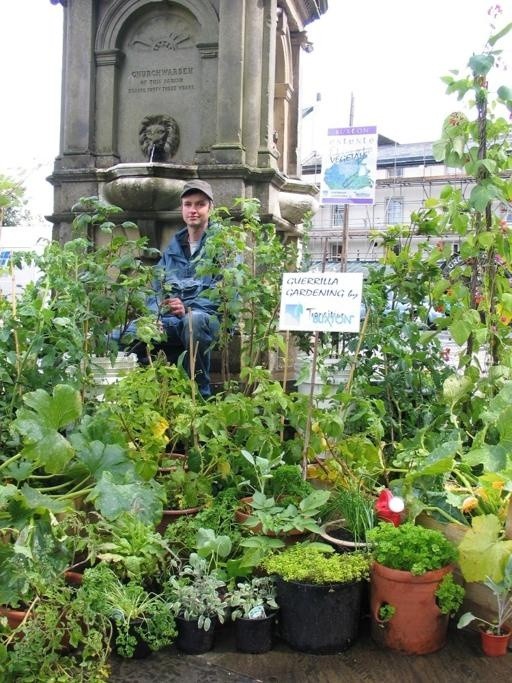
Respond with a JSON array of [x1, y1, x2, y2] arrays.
[[179, 179, 213, 201]]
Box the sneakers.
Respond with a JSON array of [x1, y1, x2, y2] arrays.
[[200, 383, 212, 397]]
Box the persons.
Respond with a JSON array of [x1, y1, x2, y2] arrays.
[[106, 178, 245, 392]]
